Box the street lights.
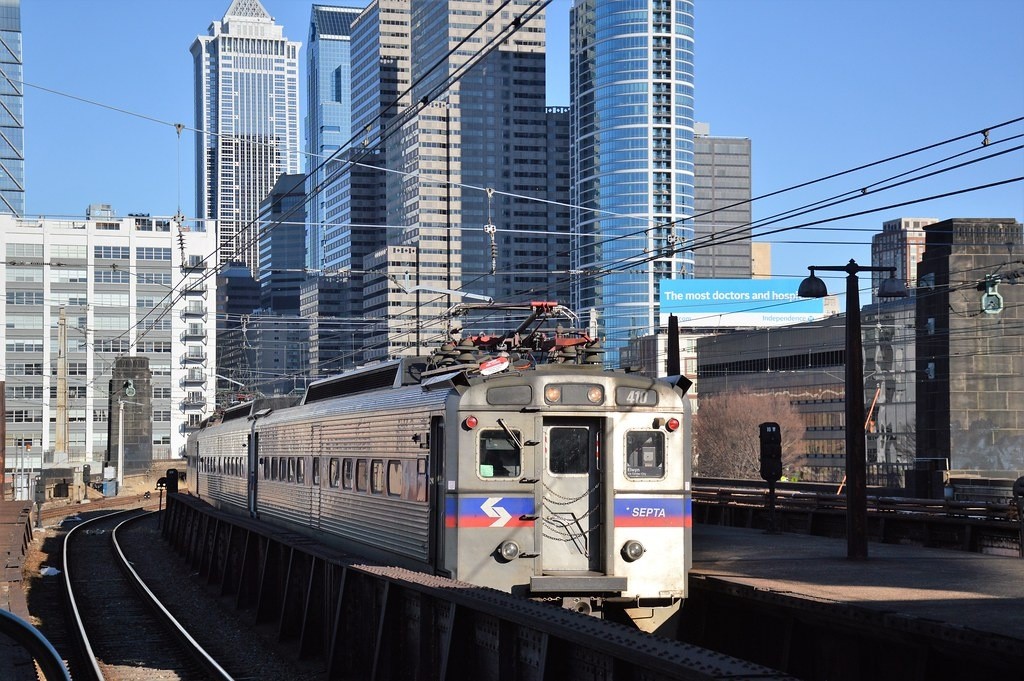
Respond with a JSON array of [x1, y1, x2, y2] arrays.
[[797, 261, 909, 568]]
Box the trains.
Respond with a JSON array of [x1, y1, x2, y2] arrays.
[[182, 299, 698, 638]]
